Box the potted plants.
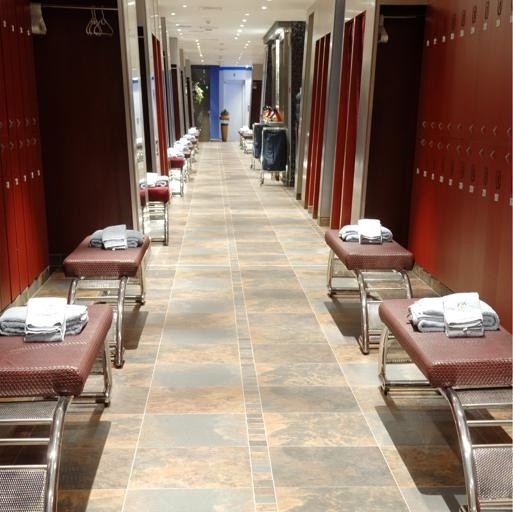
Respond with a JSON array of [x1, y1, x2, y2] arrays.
[[219, 110, 230, 121]]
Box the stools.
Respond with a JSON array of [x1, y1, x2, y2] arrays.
[[171, 127, 204, 199], [144, 174, 171, 245]]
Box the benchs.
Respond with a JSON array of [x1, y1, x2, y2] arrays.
[[237, 127, 253, 155], [61, 233, 151, 367], [323, 226, 412, 353], [0, 303, 117, 511], [376, 297, 513, 511]]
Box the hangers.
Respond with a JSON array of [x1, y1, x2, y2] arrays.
[[85, 5, 115, 38]]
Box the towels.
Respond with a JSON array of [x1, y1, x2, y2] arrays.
[[406, 288, 495, 338], [23, 296, 66, 344], [357, 218, 383, 243], [0, 304, 88, 338], [168, 127, 200, 158], [102, 223, 127, 250], [89, 229, 145, 248], [137, 171, 169, 187], [443, 292, 485, 336], [239, 124, 252, 135], [339, 223, 393, 243]]
